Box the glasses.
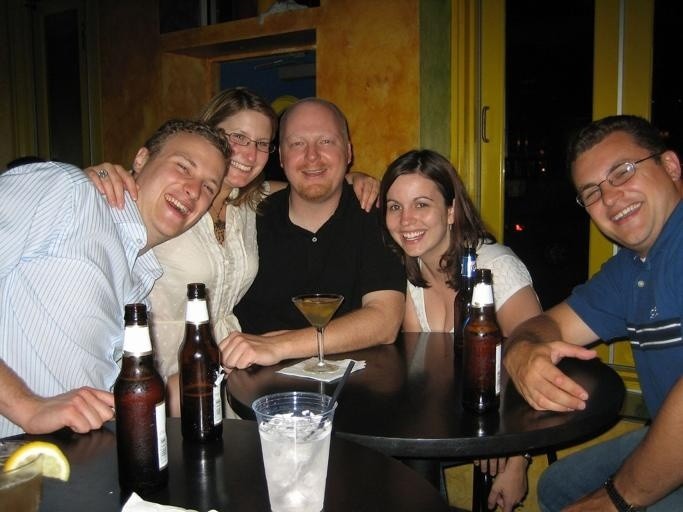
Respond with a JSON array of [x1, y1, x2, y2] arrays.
[[224, 133, 276, 154], [576, 152, 661, 208]]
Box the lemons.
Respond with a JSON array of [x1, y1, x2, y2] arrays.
[[3, 441, 69, 482]]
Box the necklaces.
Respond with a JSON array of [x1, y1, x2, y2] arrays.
[[211, 198, 228, 244]]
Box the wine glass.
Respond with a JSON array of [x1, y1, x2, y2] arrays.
[[290, 294, 344, 373]]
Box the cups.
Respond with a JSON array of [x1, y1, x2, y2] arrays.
[[0, 440, 45, 512], [249, 393, 339, 512]]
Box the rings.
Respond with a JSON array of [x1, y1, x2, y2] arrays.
[[97, 168, 108, 177]]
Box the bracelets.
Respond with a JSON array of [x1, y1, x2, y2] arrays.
[[521, 451, 533, 464], [602, 474, 648, 511]]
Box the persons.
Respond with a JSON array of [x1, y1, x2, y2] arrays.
[[82, 86, 383, 419], [499, 113, 683, 511], [214, 96, 405, 371], [1, 117, 234, 434], [378, 150, 545, 511]]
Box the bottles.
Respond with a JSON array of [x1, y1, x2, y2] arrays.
[[112, 302, 170, 495], [177, 283, 223, 454], [451, 247, 479, 391], [460, 267, 502, 417]]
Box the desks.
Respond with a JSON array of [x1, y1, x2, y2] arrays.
[[225, 330, 626, 510], [1, 417, 450, 511]]
